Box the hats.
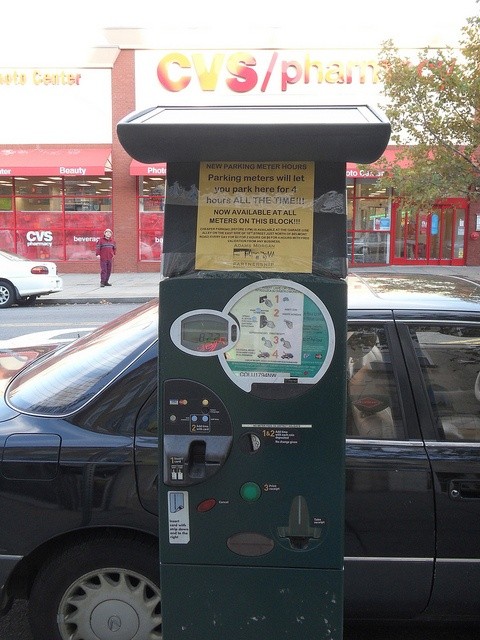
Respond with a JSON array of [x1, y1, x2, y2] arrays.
[[104, 229, 112, 234]]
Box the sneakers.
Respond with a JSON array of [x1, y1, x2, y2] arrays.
[[100, 284, 105, 287], [105, 283, 111, 286]]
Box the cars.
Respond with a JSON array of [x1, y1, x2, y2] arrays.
[[0, 247, 63, 309], [347, 229, 410, 255], [0, 273, 476, 639]]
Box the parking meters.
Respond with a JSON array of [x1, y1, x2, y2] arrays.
[[113, 98, 394, 638]]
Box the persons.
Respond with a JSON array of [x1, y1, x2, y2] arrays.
[[95, 229, 117, 287]]
